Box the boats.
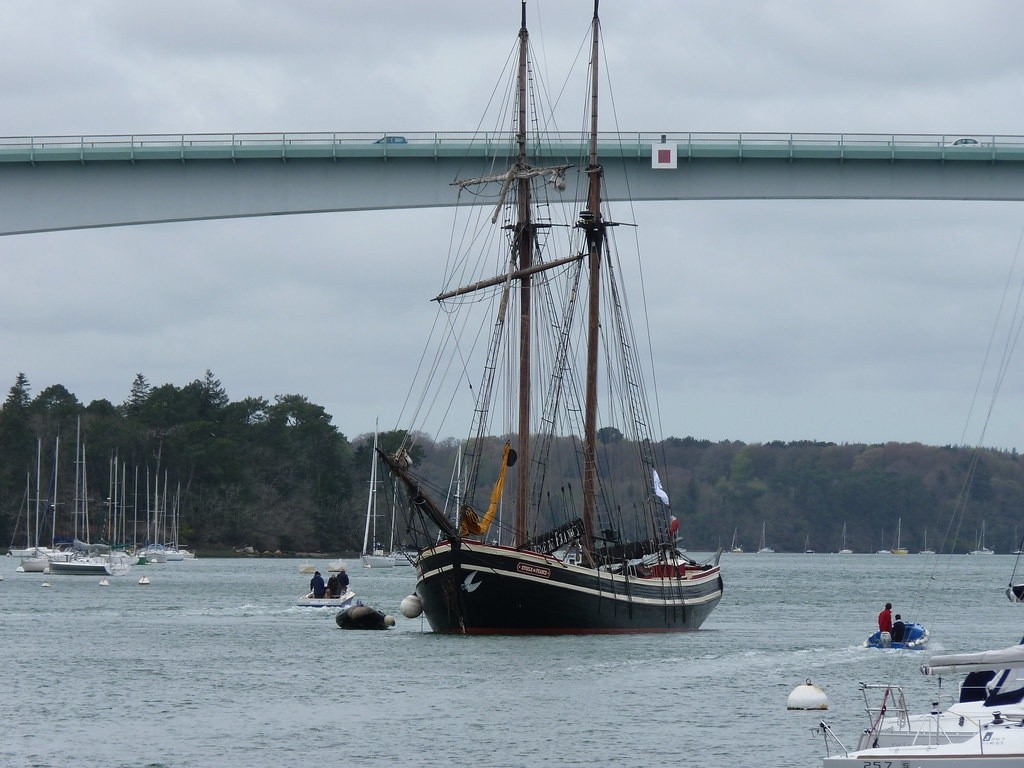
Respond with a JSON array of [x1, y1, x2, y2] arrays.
[[336, 599, 388, 631], [815, 229, 1024, 768], [296, 586, 355, 607], [862, 621, 930, 650]]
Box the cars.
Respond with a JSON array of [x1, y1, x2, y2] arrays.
[[372, 136, 408, 144], [944, 138, 982, 147]]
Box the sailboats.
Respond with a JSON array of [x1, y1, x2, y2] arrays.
[[891, 517, 910, 554], [803, 532, 815, 554], [875, 529, 892, 555], [377, 0, 726, 635], [917, 530, 935, 555], [757, 520, 775, 554], [966, 520, 994, 555], [719, 526, 744, 554], [362, 417, 411, 568], [7, 413, 195, 575], [838, 522, 853, 554]]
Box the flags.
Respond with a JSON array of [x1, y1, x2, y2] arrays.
[[653, 468, 669, 505]]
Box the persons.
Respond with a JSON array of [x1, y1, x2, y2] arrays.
[[891, 613, 906, 643], [326, 574, 341, 598], [878, 603, 892, 637], [338, 569, 349, 594], [310, 570, 326, 598]]
